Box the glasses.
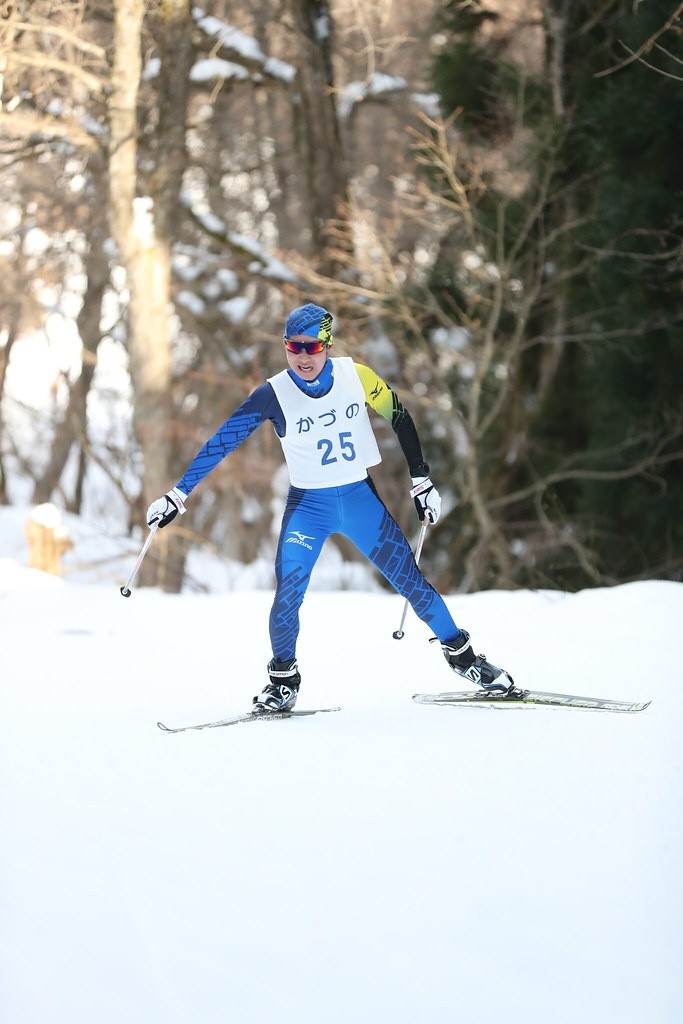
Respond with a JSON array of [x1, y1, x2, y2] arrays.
[[283, 334, 330, 355]]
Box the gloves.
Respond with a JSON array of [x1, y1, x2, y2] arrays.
[[147, 487, 188, 529], [409, 477, 441, 525]]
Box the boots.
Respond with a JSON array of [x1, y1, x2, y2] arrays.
[[441, 631, 513, 693], [253, 659, 301, 713]]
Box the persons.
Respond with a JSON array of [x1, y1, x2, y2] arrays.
[[146, 302, 514, 712]]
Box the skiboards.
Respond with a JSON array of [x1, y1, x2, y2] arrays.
[[156, 688, 654, 734]]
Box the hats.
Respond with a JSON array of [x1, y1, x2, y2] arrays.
[[285, 304, 333, 345]]
